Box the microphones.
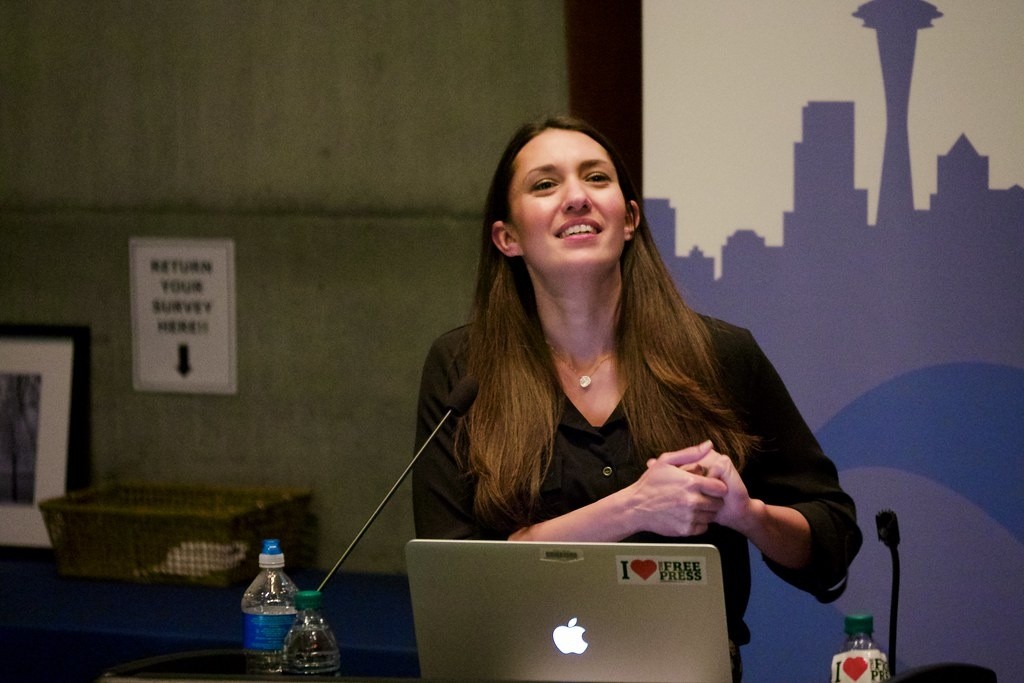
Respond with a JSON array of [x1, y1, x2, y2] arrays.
[[874, 510, 900, 676], [316, 373, 481, 593]]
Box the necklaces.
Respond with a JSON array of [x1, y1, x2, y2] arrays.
[[545, 340, 615, 388]]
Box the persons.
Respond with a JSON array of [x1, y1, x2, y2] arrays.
[[414, 116, 863, 683]]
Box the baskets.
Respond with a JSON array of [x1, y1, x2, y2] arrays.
[[40, 478, 313, 586]]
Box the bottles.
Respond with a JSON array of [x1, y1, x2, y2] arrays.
[[282, 590, 342, 677], [830, 614, 889, 683], [240, 539, 299, 674]]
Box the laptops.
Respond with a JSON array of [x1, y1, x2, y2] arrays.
[[406, 538, 733, 682]]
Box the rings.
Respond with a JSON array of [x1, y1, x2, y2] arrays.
[[696, 465, 708, 476]]
